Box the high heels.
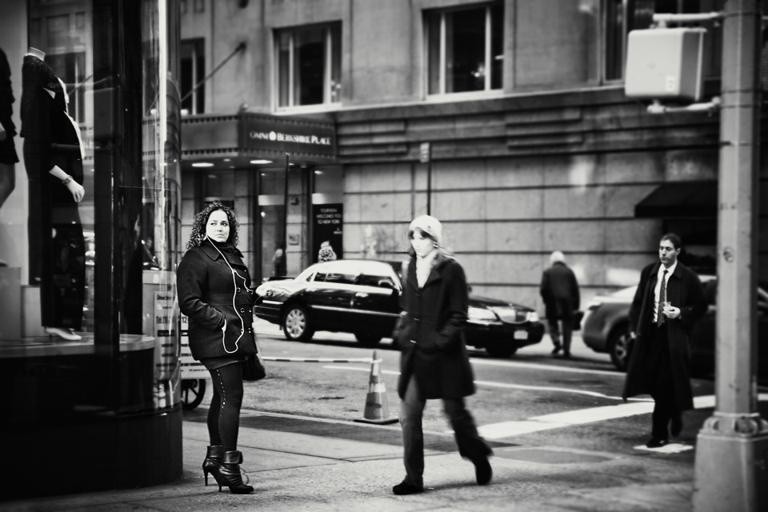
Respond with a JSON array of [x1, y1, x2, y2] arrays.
[[203, 445, 224, 485], [218, 450, 253, 494]]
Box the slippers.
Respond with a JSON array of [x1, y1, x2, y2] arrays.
[[476, 457, 491, 484]]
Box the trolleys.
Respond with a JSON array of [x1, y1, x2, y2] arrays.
[[140, 268, 214, 410]]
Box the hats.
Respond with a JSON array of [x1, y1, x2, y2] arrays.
[[410, 216, 441, 240]]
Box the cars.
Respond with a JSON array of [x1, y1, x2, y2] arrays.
[[252, 258, 548, 361], [578, 271, 768, 375]]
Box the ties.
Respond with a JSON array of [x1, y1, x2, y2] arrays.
[[657, 271, 667, 327]]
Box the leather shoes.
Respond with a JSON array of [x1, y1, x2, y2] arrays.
[[393, 475, 423, 495]]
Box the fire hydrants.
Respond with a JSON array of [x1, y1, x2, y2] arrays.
[[417, 139, 435, 217]]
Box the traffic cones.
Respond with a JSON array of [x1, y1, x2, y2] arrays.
[[351, 346, 401, 427]]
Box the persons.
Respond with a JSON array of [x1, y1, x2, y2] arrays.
[[1, 48, 19, 208], [621, 232, 709, 447], [540, 250, 580, 359], [393, 215, 493, 495], [175, 203, 266, 496], [271, 248, 284, 277], [23, 78, 86, 342]]
